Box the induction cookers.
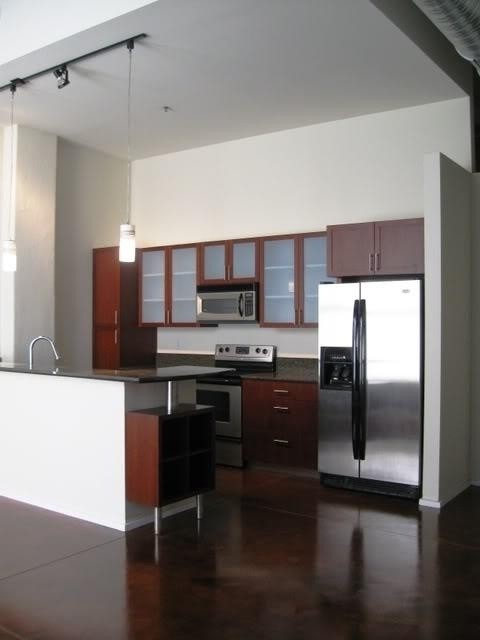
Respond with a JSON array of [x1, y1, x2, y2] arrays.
[[212, 361, 277, 377]]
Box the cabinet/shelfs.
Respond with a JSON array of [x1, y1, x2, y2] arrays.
[[242, 380, 318, 473], [326, 218, 424, 277], [92, 247, 155, 367], [197, 240, 259, 283], [142, 248, 198, 325], [259, 237, 336, 326], [124, 409, 217, 508]]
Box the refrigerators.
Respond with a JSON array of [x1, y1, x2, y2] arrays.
[[317, 279, 425, 499]]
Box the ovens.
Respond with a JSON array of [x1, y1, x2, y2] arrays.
[[195, 374, 242, 467]]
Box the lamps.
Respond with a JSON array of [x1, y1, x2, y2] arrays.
[[52, 65, 70, 90], [117, 41, 138, 268], [0, 78, 22, 273]]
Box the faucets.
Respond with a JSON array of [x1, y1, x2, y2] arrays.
[[27, 335, 63, 371]]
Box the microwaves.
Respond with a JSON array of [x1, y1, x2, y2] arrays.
[[196, 291, 258, 324]]
